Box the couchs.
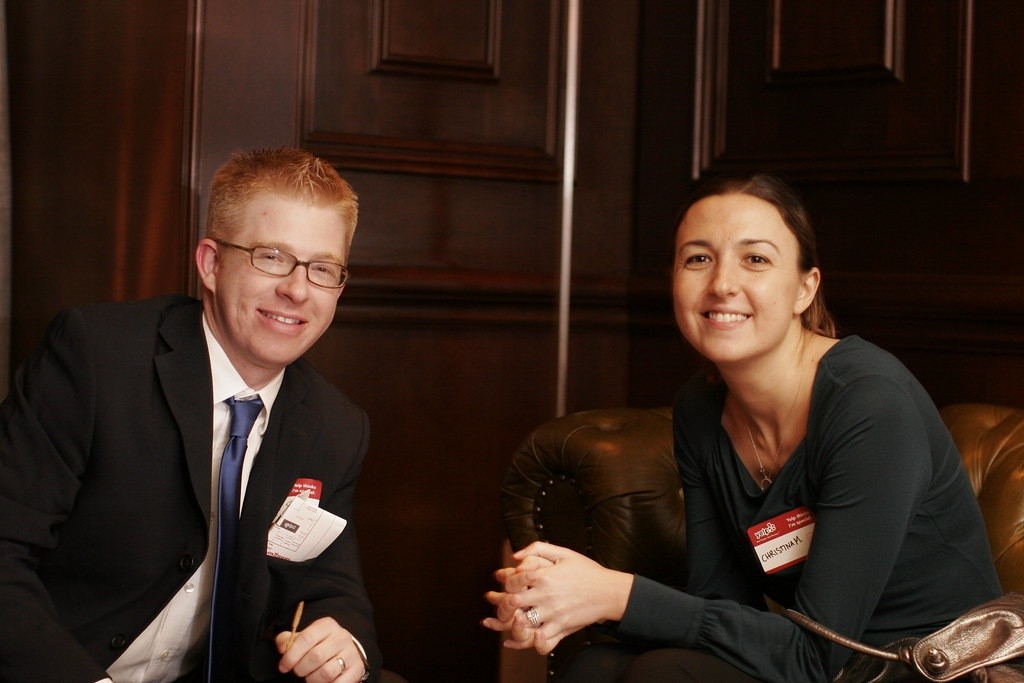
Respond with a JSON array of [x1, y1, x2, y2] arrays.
[[497, 400, 1024, 683]]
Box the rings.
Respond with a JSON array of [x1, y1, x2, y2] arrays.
[[335, 657, 346, 674], [526, 607, 541, 628]]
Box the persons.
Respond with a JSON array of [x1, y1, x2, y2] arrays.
[[483, 163, 1007, 683], [0, 138, 383, 683]]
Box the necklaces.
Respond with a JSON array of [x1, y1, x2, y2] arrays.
[[745, 356, 807, 492]]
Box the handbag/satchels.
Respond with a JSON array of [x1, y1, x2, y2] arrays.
[[773, 591, 1024, 683]]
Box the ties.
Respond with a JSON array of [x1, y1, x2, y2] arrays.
[[208, 397, 265, 683]]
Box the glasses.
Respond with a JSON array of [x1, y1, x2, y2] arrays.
[[215, 238, 351, 288]]
